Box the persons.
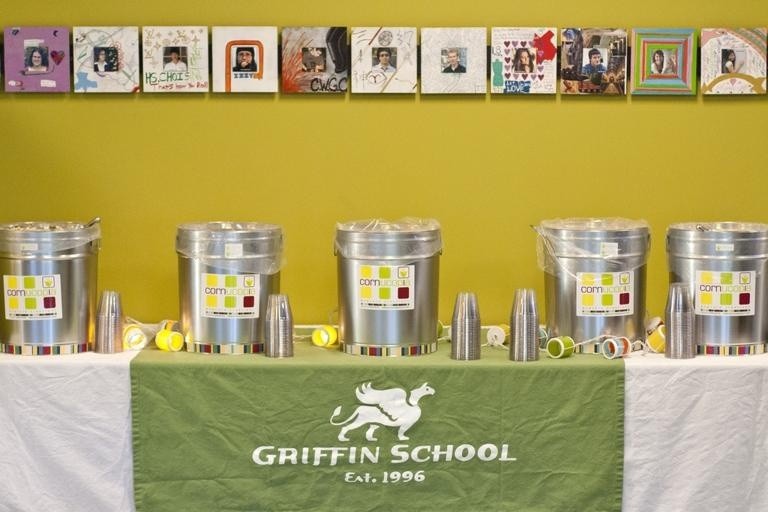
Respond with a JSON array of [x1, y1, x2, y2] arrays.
[[164, 49, 187, 72], [372, 48, 396, 72], [302, 48, 326, 72], [722, 51, 745, 74], [232, 47, 257, 73], [441, 50, 466, 74], [94, 49, 111, 72], [651, 50, 673, 75], [26, 48, 47, 72], [581, 49, 608, 74], [512, 48, 535, 74]]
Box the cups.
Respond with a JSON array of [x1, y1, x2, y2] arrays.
[[662, 286, 699, 359], [263, 294, 296, 360], [447, 290, 481, 361], [92, 290, 123, 357], [506, 288, 541, 363]]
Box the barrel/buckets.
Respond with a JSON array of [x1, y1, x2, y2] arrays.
[[0, 220, 101, 357], [529, 216, 652, 354], [174, 219, 284, 355], [333, 218, 443, 359], [664, 220, 768, 356]]
